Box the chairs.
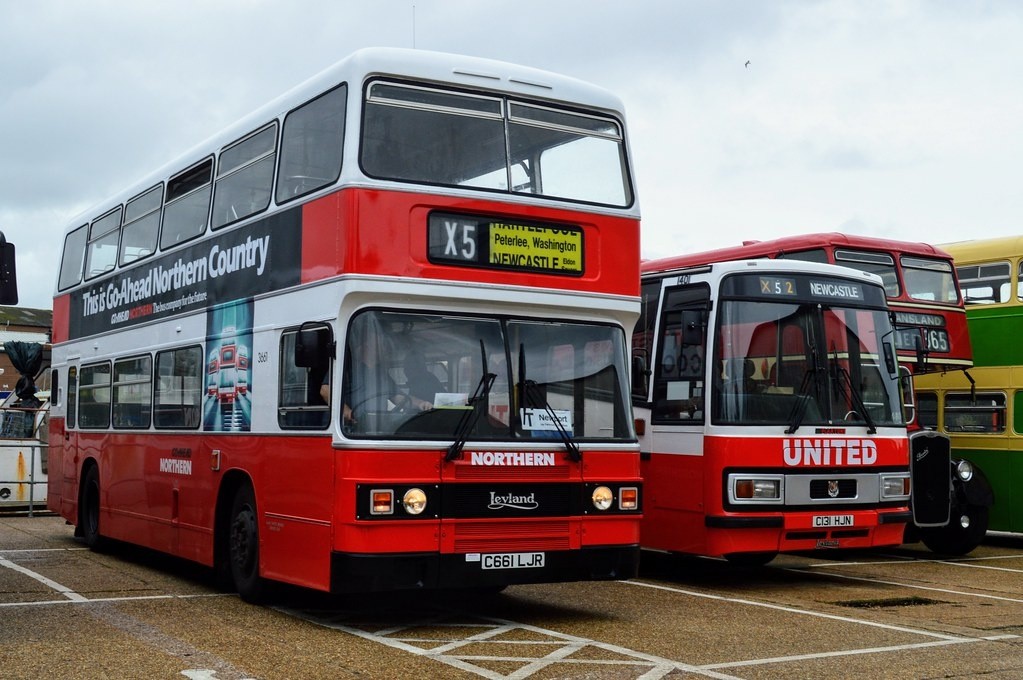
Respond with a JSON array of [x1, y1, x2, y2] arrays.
[[231, 200, 252, 219], [90, 248, 154, 278]]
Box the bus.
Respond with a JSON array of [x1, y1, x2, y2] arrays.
[[642, 231, 1023, 559], [48, 44, 653, 608], [400, 257, 931, 582]]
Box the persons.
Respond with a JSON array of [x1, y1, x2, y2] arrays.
[[320, 325, 446, 432]]
